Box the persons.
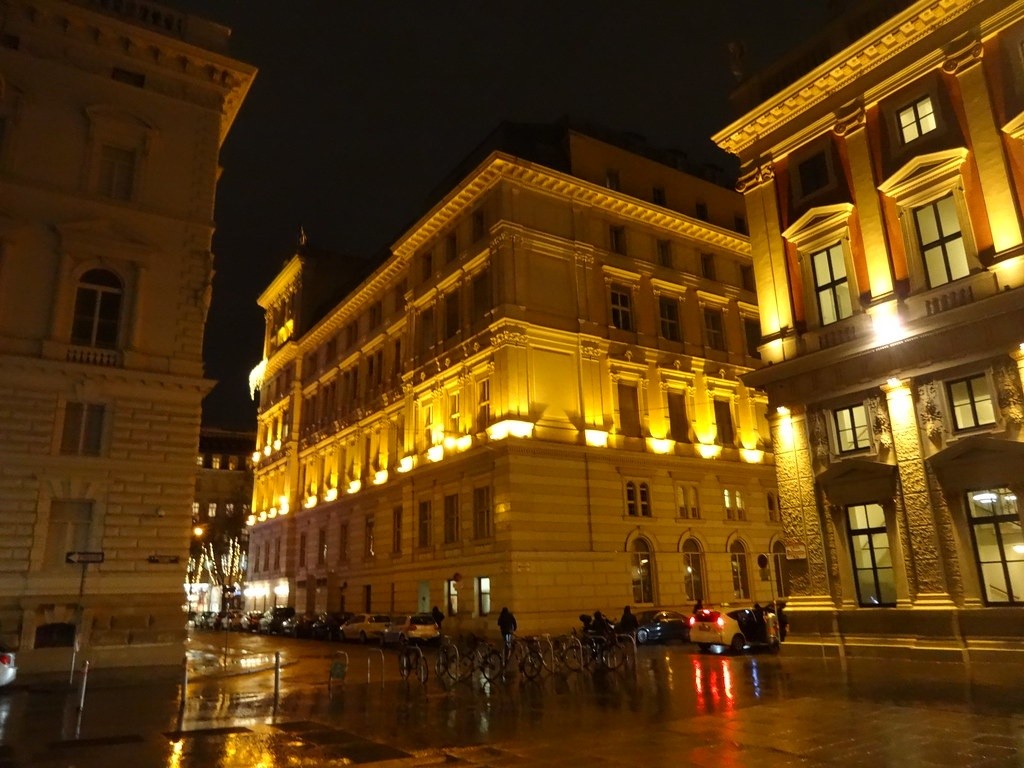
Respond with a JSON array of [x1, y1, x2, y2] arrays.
[[693, 599, 703, 614], [592, 611, 607, 661], [498, 606, 517, 647], [432, 606, 445, 631], [621, 605, 638, 648]]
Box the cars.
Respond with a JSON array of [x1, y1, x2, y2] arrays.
[[311, 610, 356, 641], [0, 639, 19, 687], [282, 614, 330, 640], [385, 613, 441, 646], [192, 610, 264, 631], [339, 612, 395, 646], [629, 609, 689, 644], [685, 605, 780, 653]]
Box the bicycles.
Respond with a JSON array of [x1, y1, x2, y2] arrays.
[[544, 616, 628, 674], [434, 635, 460, 685], [395, 638, 429, 685], [455, 633, 504, 681], [499, 637, 543, 680]]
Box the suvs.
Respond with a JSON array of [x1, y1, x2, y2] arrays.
[[258, 605, 296, 636]]
